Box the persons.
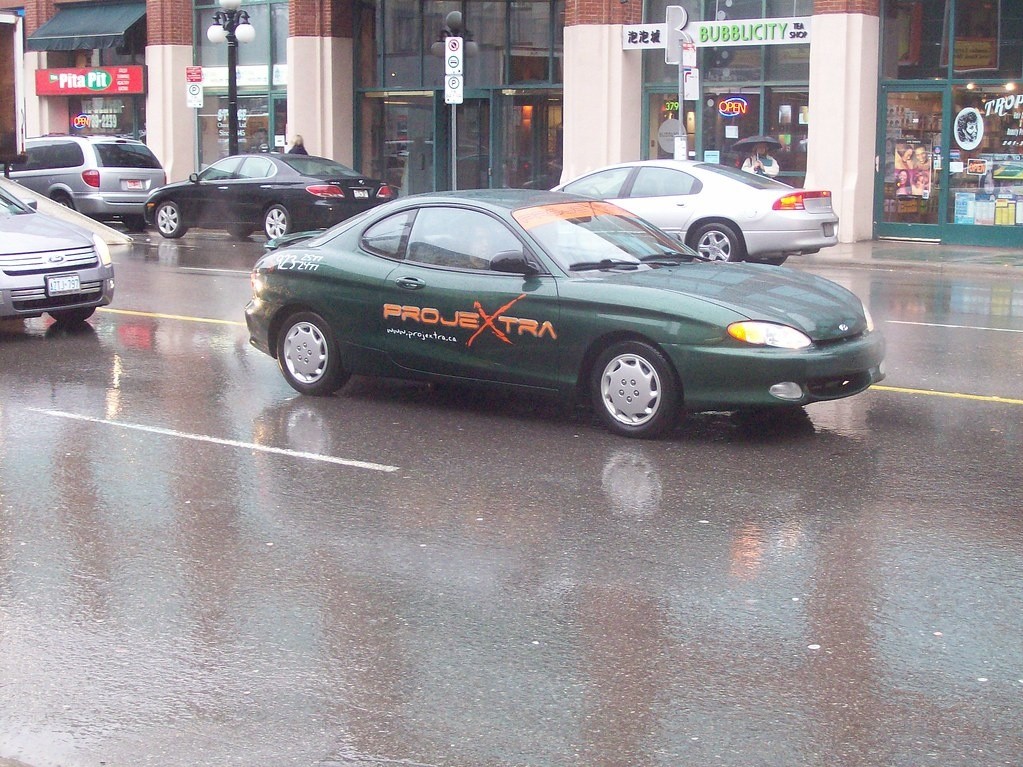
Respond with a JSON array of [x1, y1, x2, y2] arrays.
[[455, 222, 509, 270], [958, 112, 978, 143], [288, 135, 308, 155], [895, 144, 930, 194], [975, 171, 1000, 201], [742, 143, 779, 177]]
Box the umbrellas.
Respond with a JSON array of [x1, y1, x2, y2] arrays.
[[731, 136, 782, 169]]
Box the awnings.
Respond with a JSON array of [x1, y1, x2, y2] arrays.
[[26, 0, 146, 52]]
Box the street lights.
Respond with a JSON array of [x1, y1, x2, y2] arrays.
[[206, 0, 256, 157], [432, 11, 479, 191]]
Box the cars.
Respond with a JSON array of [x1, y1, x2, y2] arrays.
[[244, 186, 889, 438], [0, 187, 115, 323], [143, 153, 393, 241], [550, 158, 840, 266], [384, 138, 563, 191]]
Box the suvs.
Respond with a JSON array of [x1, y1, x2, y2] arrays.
[[0, 131, 168, 231]]
